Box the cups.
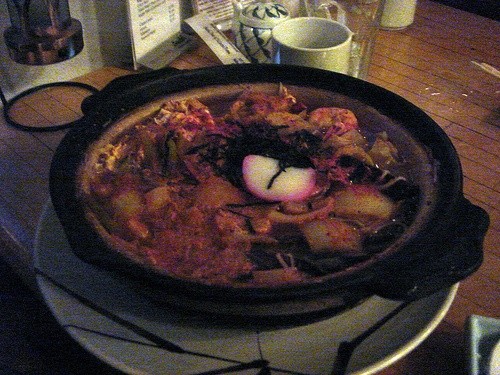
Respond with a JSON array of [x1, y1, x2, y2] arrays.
[[272, 17, 351, 74], [299, 0, 384, 80], [379, 0, 416, 32]]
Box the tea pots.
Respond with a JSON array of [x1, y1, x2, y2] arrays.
[[227, 0, 291, 64]]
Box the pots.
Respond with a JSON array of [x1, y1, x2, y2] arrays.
[[50, 62, 489, 332]]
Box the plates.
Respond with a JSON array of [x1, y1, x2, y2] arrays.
[[32, 197, 460, 375]]
